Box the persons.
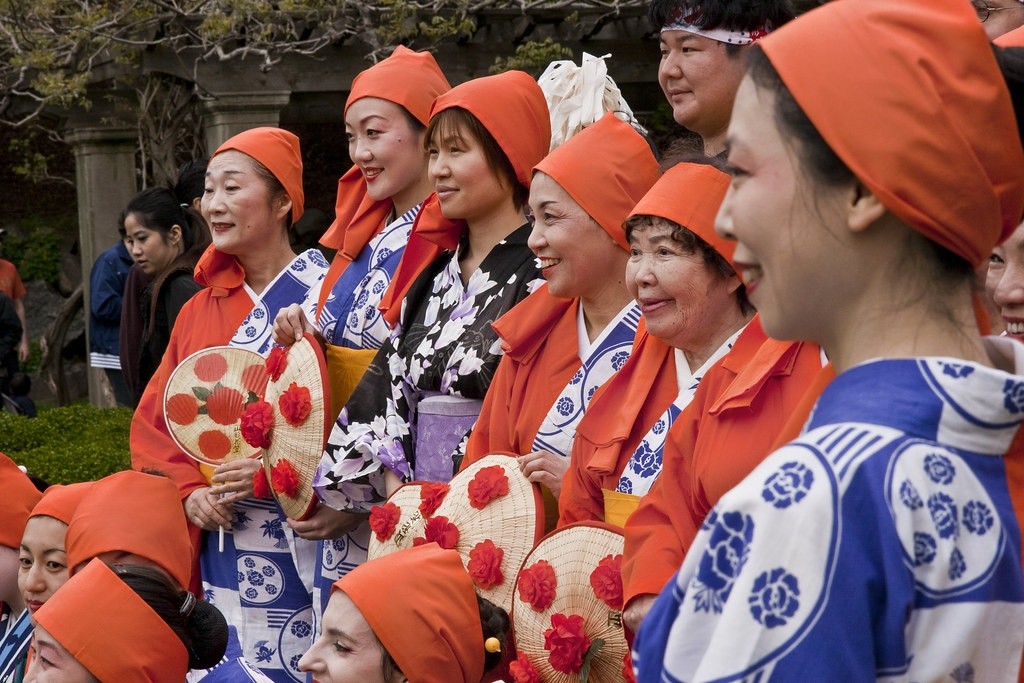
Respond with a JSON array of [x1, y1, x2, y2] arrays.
[[129, 125, 328, 683], [272, 44, 452, 639], [0, 450, 51, 683], [971, 1, 1023, 47], [621, 0, 1024, 682], [66, 471, 194, 590], [509, 154, 760, 681], [983, 214, 1024, 558], [314, 68, 550, 562], [87, 159, 208, 410], [17, 480, 95, 683], [295, 542, 509, 683], [653, 0, 831, 162], [0, 228, 36, 417], [428, 113, 667, 606], [20, 558, 229, 683]]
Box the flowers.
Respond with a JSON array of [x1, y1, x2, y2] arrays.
[[244, 346, 633, 682]]
